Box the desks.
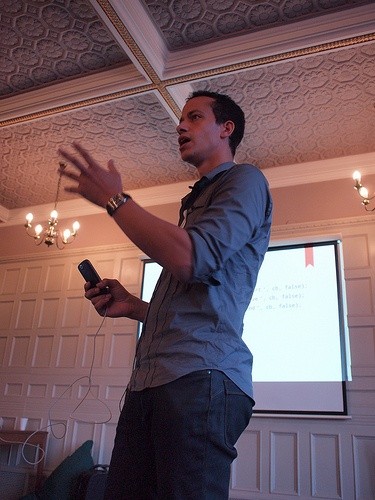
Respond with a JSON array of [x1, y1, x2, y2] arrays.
[[0, 429, 48, 489]]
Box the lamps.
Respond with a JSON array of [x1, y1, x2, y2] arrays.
[[352, 170, 375, 212], [25, 164, 80, 250]]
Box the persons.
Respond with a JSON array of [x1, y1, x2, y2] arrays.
[[57, 91, 274, 500]]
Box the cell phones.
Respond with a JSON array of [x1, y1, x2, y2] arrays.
[[78, 259, 108, 296]]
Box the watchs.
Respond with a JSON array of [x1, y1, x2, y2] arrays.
[[104, 191, 131, 217]]
